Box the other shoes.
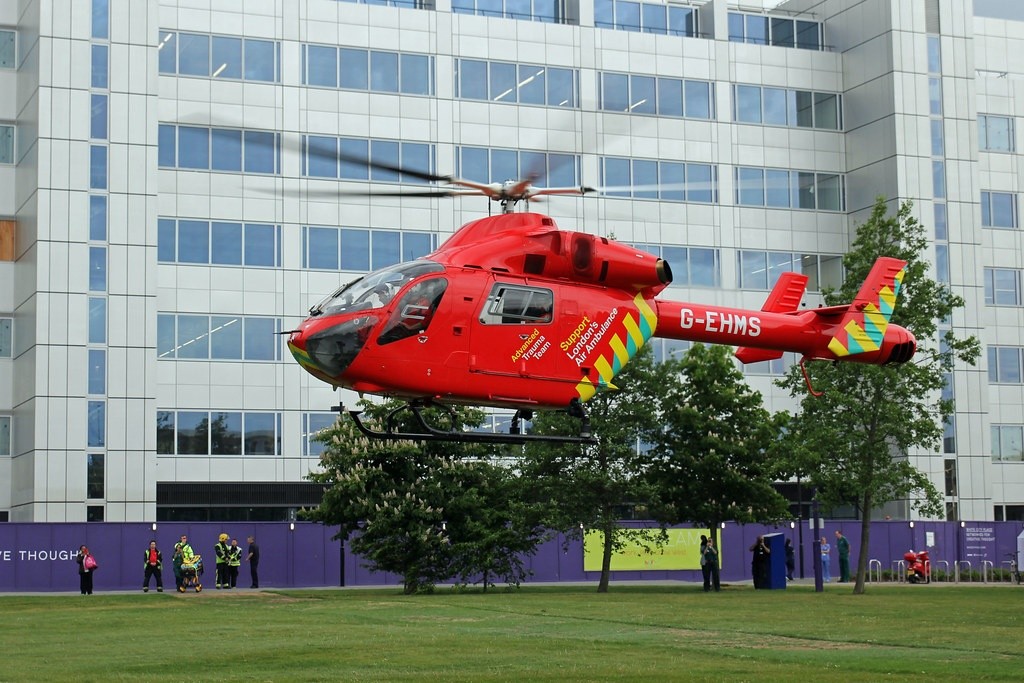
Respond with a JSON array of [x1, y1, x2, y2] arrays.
[[223, 585, 231, 588]]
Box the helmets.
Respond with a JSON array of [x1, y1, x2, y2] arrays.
[[219, 533, 231, 541]]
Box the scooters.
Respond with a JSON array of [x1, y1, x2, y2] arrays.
[[904, 549, 932, 584]]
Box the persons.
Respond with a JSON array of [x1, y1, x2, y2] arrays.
[[143, 540, 163, 592], [76, 544, 98, 595], [748, 535, 770, 590], [214, 533, 232, 589], [373, 277, 433, 335], [835, 530, 850, 583], [821, 536, 831, 583], [700, 535, 722, 593], [522, 289, 552, 323], [228, 538, 242, 589], [245, 535, 259, 588], [785, 537, 795, 581], [172, 534, 196, 592]]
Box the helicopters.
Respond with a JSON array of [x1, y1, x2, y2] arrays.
[[183, 36, 919, 446]]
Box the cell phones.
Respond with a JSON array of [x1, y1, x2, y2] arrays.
[[84, 548, 87, 554]]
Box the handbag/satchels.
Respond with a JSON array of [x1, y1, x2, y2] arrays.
[[85, 554, 96, 570]]
